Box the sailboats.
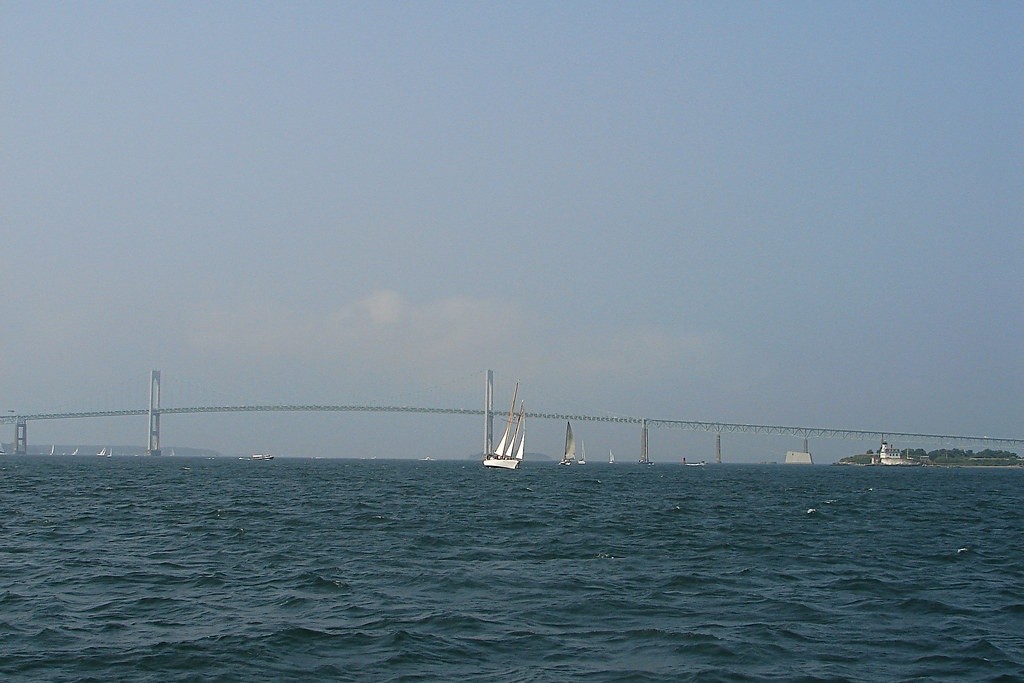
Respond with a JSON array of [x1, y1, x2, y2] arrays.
[[578, 440, 586, 465], [608, 450, 616, 465], [558, 422, 576, 466], [483, 381, 526, 470]]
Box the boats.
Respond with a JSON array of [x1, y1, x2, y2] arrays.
[[684, 460, 707, 467], [251, 454, 274, 461], [879, 440, 921, 466]]
